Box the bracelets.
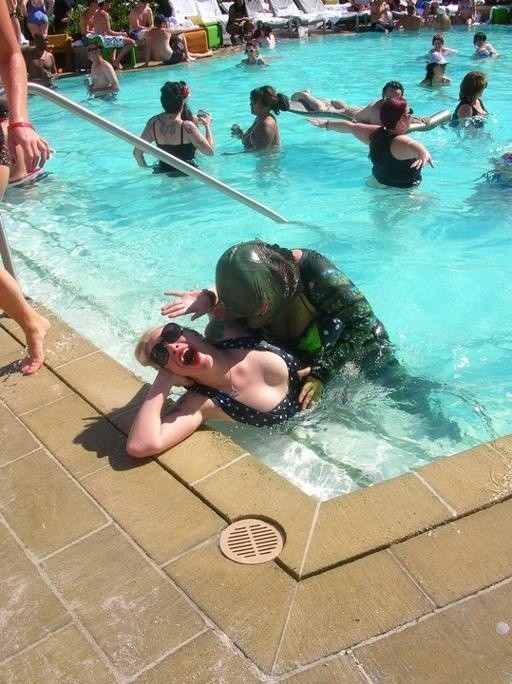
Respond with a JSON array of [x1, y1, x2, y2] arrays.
[[203, 288, 218, 302], [5, 120, 37, 130]]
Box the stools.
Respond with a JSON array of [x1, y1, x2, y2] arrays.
[[204, 24, 224, 49], [181, 26, 208, 53]]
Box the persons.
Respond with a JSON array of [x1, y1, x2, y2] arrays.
[[79, 0, 100, 47], [202, 236, 410, 416], [93, 0, 136, 71], [427, 33, 458, 56], [6, 0, 21, 43], [488, 153, 511, 195], [25, 32, 58, 90], [0, 1, 51, 378], [225, 0, 256, 47], [130, 81, 215, 177], [134, 12, 215, 71], [230, 84, 292, 152], [290, 80, 433, 127], [471, 31, 497, 61], [84, 41, 122, 103], [453, 70, 494, 130], [127, 0, 155, 45], [124, 286, 311, 461], [365, 96, 436, 188], [18, 0, 51, 43], [183, 97, 200, 130], [420, 50, 453, 87], [52, 0, 78, 36], [254, 23, 277, 50], [235, 41, 271, 66], [368, 0, 475, 30]]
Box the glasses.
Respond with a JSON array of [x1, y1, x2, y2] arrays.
[[148, 321, 182, 365], [484, 81, 489, 89], [402, 108, 414, 116], [245, 49, 255, 54]]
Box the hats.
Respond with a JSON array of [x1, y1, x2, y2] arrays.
[[154, 13, 170, 23], [426, 52, 449, 65], [88, 34, 104, 50], [216, 241, 291, 329]]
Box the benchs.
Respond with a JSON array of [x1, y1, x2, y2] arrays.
[[72, 44, 136, 70]]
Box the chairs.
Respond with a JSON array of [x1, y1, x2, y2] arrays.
[[195, 0, 258, 45], [44, 34, 72, 72], [167, 0, 230, 51], [219, 2, 293, 40], [244, 0, 327, 39], [295, 0, 371, 33], [429, 0, 458, 14], [269, 0, 360, 33]]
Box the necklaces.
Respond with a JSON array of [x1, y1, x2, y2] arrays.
[[209, 352, 241, 400]]
